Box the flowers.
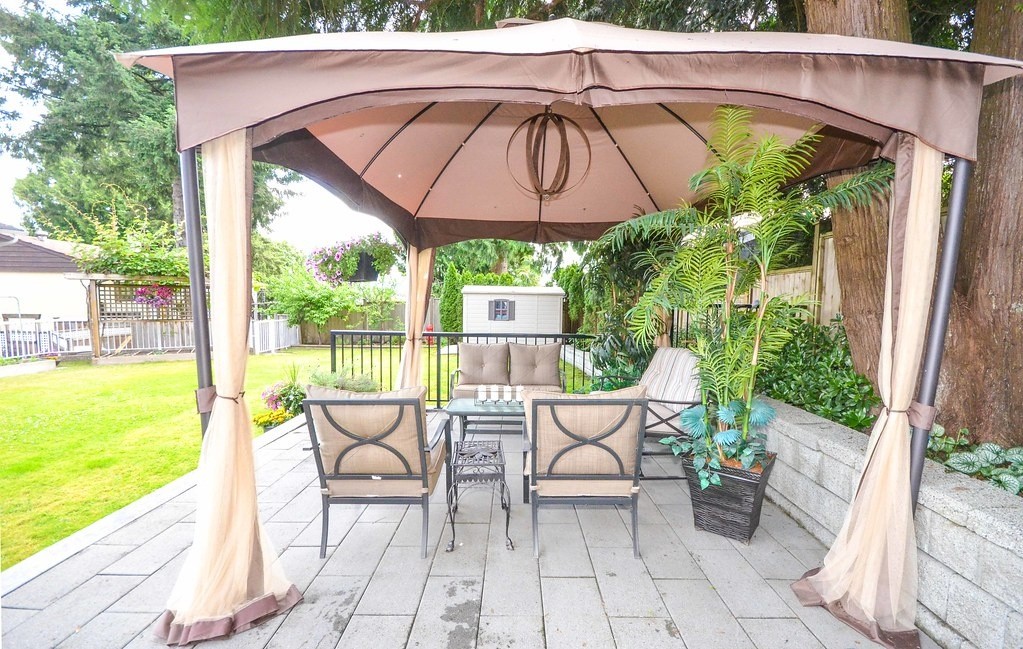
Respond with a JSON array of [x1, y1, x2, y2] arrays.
[[304, 232, 402, 290], [260, 381, 283, 411], [133, 284, 172, 309], [252, 408, 295, 428]]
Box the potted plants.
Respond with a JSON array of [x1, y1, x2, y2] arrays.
[[576, 104, 895, 546]]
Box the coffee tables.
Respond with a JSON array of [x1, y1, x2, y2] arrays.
[[442, 397, 526, 442]]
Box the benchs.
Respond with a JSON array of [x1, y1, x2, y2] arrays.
[[449, 369, 567, 431], [597, 347, 712, 480]]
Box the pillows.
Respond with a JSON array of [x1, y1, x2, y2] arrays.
[[521, 385, 647, 443], [458, 342, 509, 386], [304, 384, 430, 470], [508, 342, 561, 386]]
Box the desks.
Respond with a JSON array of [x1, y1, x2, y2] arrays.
[[446, 440, 514, 552]]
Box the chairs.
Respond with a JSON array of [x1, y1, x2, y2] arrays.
[[302, 397, 452, 558], [523, 398, 650, 559]]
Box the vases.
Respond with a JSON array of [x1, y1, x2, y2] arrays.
[[342, 251, 380, 282], [262, 426, 274, 433]]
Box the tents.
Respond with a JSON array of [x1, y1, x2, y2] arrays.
[[115, 17, 1023, 649]]
